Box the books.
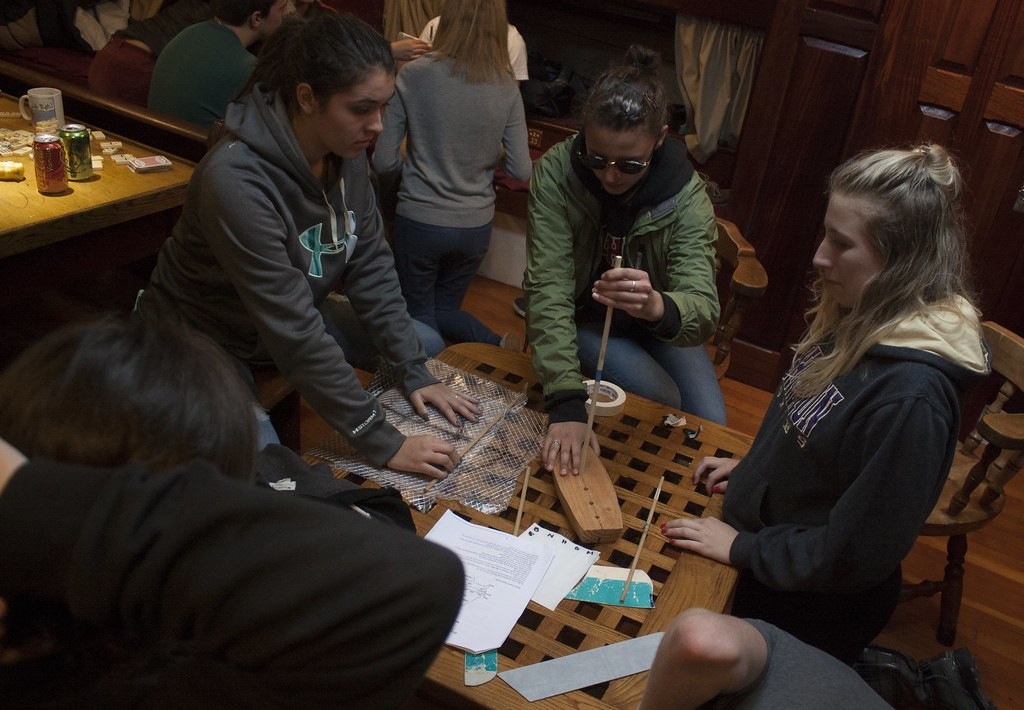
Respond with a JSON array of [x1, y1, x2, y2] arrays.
[[421, 508, 556, 655]]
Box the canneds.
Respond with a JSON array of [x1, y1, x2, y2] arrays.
[[58, 124, 93, 181], [33, 135, 69, 194]]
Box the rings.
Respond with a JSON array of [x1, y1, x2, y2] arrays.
[[552, 440, 560, 445], [630, 280, 636, 292], [455, 393, 459, 398]]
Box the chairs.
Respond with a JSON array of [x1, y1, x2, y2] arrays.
[[900, 322, 1024, 646], [512, 215, 769, 384]]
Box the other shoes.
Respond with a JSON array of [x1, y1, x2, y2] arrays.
[[499, 332, 519, 351], [513, 297, 526, 318]]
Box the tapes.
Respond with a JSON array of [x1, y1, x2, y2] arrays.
[[582, 379, 626, 416]]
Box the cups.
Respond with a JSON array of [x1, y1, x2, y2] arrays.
[[19, 88, 65, 135]]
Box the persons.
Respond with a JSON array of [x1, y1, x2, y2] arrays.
[[635, 607, 892, 710], [0, 314, 466, 710], [134, 6, 484, 480], [527, 67, 726, 478], [0, 0, 527, 196], [660, 144, 994, 659], [374, 0, 532, 350]]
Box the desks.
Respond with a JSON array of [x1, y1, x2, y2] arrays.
[[1, 88, 200, 264], [299, 341, 756, 710]]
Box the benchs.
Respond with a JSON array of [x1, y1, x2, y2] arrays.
[[0, 59, 228, 164]]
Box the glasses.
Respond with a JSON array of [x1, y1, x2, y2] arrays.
[[578, 132, 662, 174]]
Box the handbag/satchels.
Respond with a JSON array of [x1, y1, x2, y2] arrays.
[[519, 60, 577, 117]]
